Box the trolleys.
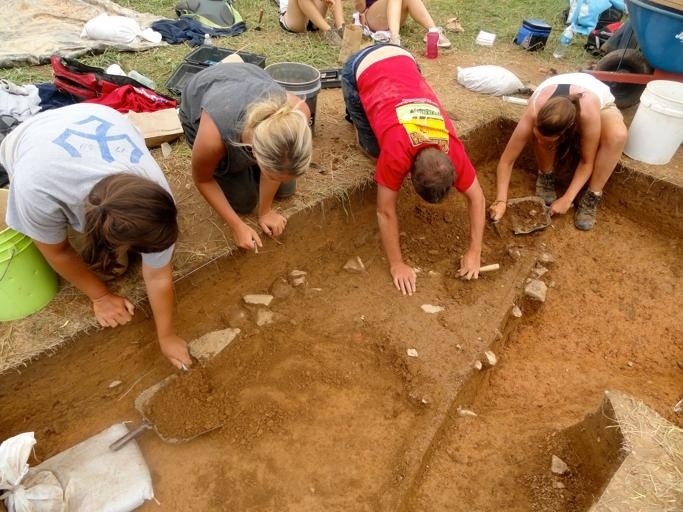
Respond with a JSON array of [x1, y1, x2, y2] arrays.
[[580, 0, 682, 110]]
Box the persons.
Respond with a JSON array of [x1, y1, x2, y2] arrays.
[[178, 62, 312, 253], [0, 102, 193, 371], [341, 41, 486, 296], [279, 0, 347, 46], [353, 0, 452, 48], [489, 72, 628, 231]]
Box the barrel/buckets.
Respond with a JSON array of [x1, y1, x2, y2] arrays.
[[623, 80, 683, 165], [264, 61, 321, 139], [0, 189, 59, 321]]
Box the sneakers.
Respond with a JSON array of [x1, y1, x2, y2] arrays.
[[423, 27, 452, 47], [574, 188, 603, 231], [325, 23, 349, 45], [389, 34, 401, 46], [535, 170, 557, 204]]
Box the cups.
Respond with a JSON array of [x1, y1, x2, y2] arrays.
[[427, 31, 439, 58]]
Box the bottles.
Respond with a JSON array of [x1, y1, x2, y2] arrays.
[[128, 70, 156, 92], [552, 27, 574, 59], [204, 34, 212, 46]]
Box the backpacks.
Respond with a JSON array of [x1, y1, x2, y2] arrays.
[[175, 0, 246, 38], [49, 54, 144, 98]]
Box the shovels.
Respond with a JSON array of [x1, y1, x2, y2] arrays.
[[507, 193, 573, 236], [255, 10, 264, 31], [110, 370, 228, 452]]
